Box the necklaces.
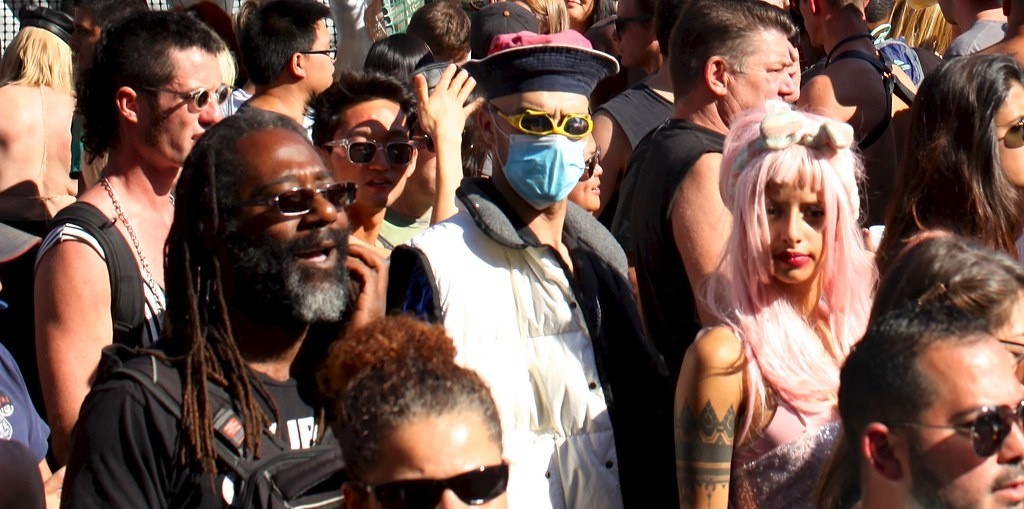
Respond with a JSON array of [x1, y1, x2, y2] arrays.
[[100, 171, 175, 327]]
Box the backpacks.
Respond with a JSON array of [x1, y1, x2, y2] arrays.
[[93, 335, 349, 509]]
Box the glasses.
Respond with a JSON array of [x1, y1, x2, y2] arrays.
[[884, 400, 1024, 459], [284, 47, 337, 66], [579, 145, 601, 182], [134, 85, 229, 111], [483, 101, 593, 140], [995, 118, 1024, 149], [222, 181, 358, 217], [351, 461, 509, 509], [412, 126, 470, 151], [614, 17, 654, 33], [323, 139, 419, 168]]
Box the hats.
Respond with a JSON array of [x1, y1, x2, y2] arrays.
[[472, 2, 538, 58], [18, 2, 75, 47], [187, 1, 242, 65], [461, 29, 619, 100], [401, 61, 479, 128], [0, 223, 42, 261]]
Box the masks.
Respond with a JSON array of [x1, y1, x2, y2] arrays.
[[486, 112, 596, 210]]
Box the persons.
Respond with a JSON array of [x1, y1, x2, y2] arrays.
[[387, 31, 678, 509], [0, 0, 1024, 509], [60, 111, 389, 509], [672, 101, 880, 509], [320, 316, 509, 509]]
